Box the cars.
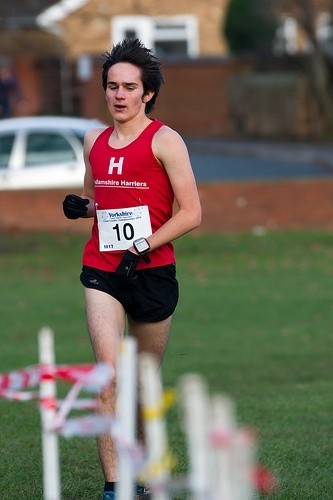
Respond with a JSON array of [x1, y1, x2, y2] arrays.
[[0, 117, 116, 190]]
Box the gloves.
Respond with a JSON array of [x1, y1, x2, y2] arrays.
[[115, 249, 138, 286], [62, 194, 90, 219]]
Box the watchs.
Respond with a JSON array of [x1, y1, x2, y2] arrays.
[[132, 237, 152, 264]]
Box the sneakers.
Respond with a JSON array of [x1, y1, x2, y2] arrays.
[[101, 491, 117, 500]]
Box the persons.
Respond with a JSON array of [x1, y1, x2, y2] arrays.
[[62, 36, 202, 500]]
